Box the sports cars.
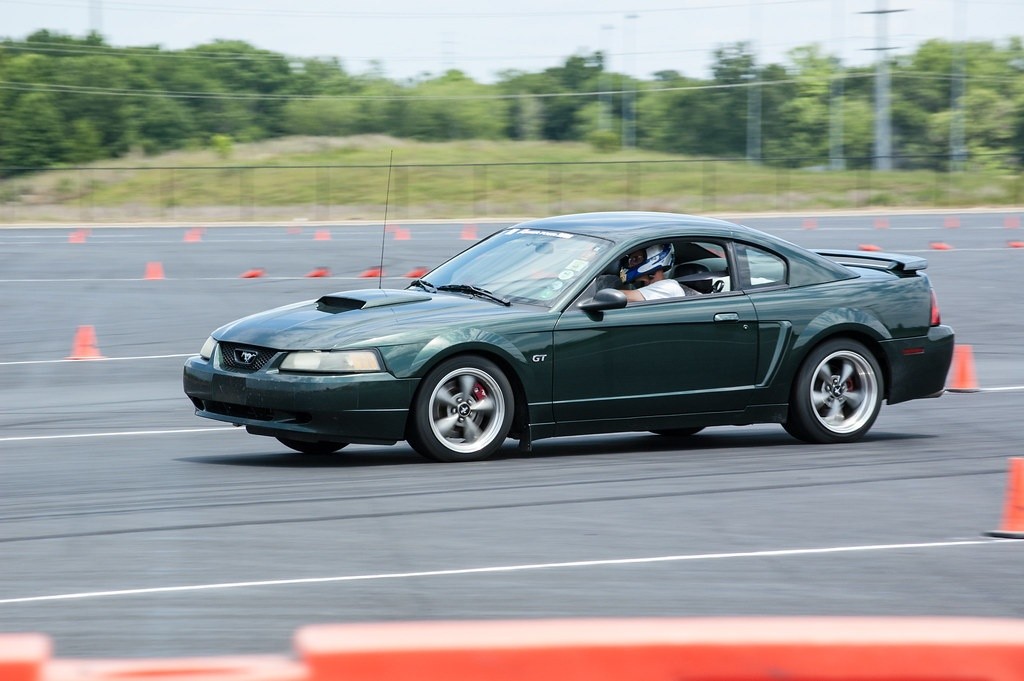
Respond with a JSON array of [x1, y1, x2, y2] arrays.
[[181, 208, 955, 463]]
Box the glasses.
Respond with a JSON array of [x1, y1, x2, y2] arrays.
[[628, 255, 644, 265]]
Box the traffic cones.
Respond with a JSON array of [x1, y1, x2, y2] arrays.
[[68, 325, 103, 358], [947, 343, 979, 393], [984, 456, 1024, 538]]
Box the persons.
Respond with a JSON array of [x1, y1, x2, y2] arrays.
[[608, 241, 685, 302]]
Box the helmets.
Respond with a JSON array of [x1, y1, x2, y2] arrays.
[[620, 242, 675, 284]]
